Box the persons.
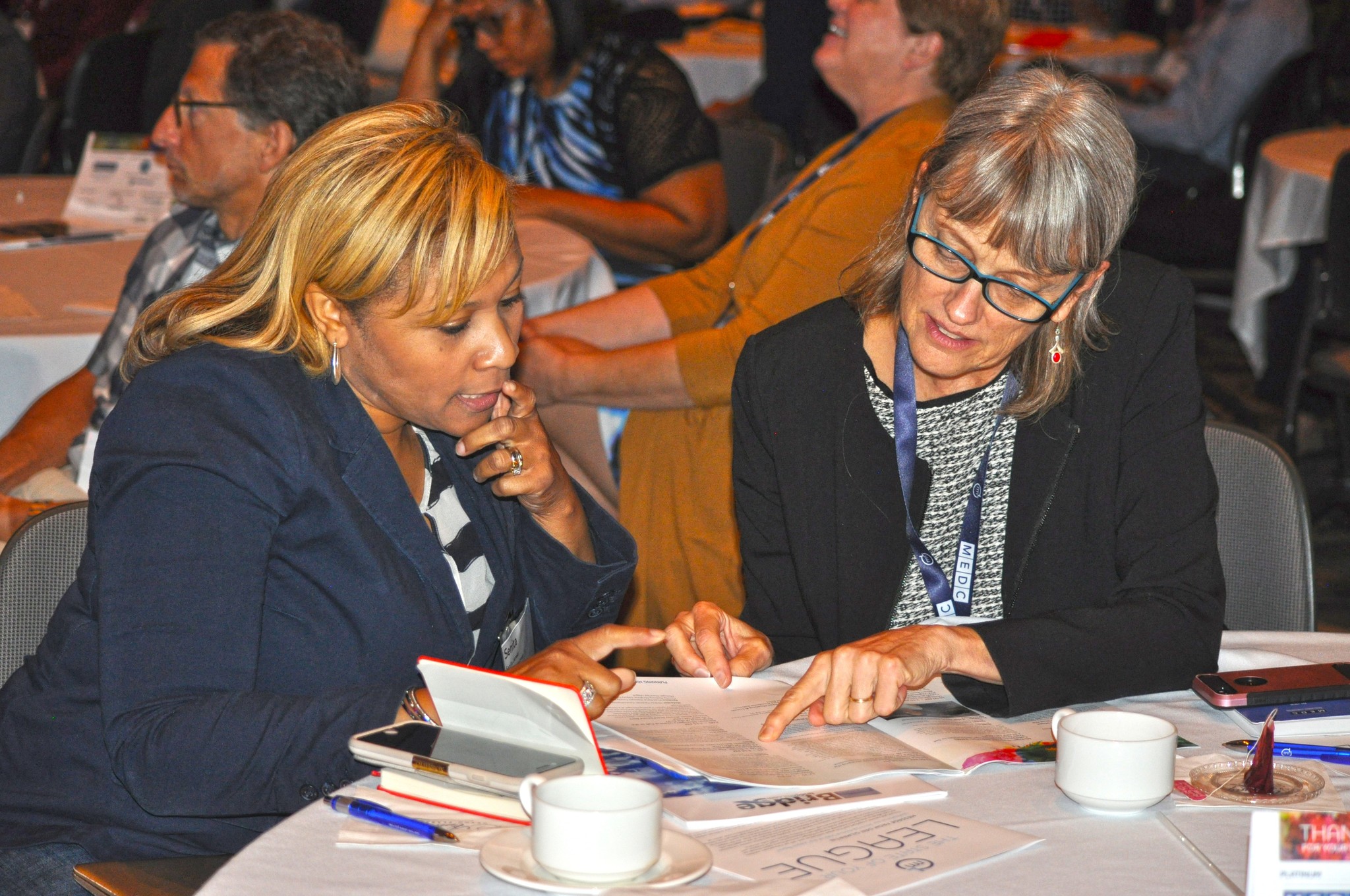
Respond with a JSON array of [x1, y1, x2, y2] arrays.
[[0, 0, 1348, 896]]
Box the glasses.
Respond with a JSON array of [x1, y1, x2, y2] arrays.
[[905, 187, 1089, 324], [174, 89, 257, 127], [451, 10, 536, 35]]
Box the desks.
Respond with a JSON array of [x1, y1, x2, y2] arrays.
[[1231, 127, 1350, 380], [192, 632, 1349, 896], [660, 18, 1156, 111], [0, 176, 627, 464]]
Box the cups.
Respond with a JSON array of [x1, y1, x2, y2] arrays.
[[1050, 707, 1177, 811], [520, 774, 663, 882]]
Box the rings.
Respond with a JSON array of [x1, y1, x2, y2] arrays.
[[501, 441, 524, 474], [578, 681, 598, 707], [848, 692, 874, 703]]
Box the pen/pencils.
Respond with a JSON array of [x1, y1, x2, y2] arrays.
[[1222, 738, 1350, 764], [320, 787, 458, 846]]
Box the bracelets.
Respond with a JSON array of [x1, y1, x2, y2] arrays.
[[405, 685, 440, 727], [400, 700, 422, 721]]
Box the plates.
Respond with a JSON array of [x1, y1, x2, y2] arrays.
[[480, 827, 712, 894]]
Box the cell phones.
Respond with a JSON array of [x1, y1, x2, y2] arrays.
[[349, 720, 586, 793], [1191, 663, 1350, 709]]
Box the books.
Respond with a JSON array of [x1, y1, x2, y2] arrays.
[[1245, 807, 1349, 896], [376, 655, 608, 826], [604, 751, 950, 829], [684, 804, 1049, 896], [597, 653, 1060, 788], [1222, 699, 1350, 739]]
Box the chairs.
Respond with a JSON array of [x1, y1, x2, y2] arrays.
[[1206, 426, 1317, 635], [1244, 50, 1327, 174], [713, 118, 789, 237], [1283, 144, 1349, 446], [0, 497, 89, 686]]
